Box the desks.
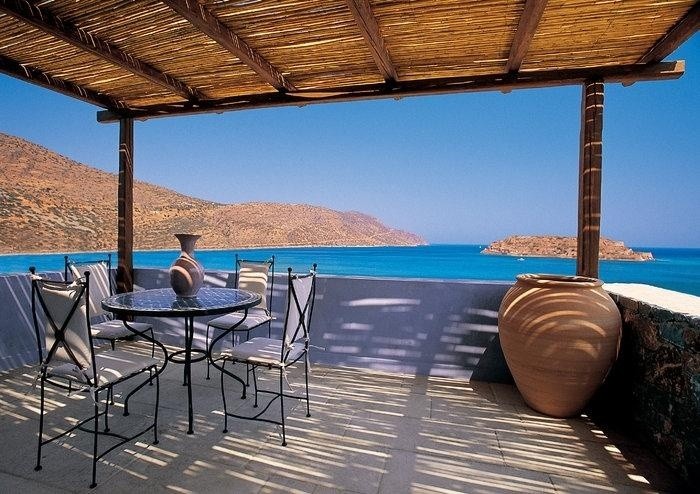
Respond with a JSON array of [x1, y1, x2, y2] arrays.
[[101, 288, 262, 435]]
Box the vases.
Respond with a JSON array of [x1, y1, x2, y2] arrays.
[[497, 272, 622, 419], [169, 234, 205, 297]]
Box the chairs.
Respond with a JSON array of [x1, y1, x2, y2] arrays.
[[64, 255, 154, 405], [221, 263, 317, 446], [29, 266, 159, 489], [206, 254, 274, 386]]
